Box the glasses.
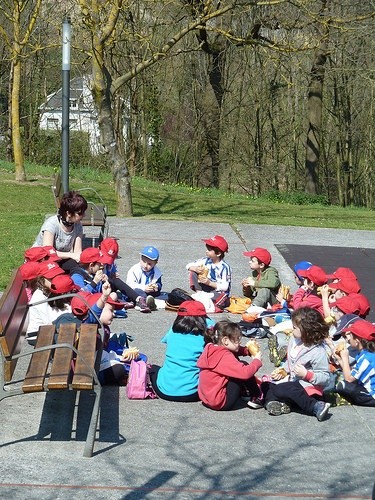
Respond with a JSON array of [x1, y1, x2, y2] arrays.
[[69, 210, 84, 216]]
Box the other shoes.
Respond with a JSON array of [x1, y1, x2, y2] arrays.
[[146, 295, 157, 311]]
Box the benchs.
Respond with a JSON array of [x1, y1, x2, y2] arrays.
[[0, 269, 102, 457], [50, 171, 109, 241]]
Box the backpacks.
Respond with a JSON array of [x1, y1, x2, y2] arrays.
[[126, 359, 159, 400]]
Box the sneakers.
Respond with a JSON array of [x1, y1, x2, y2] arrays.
[[241, 388, 251, 400], [247, 395, 264, 409], [313, 401, 330, 422], [134, 295, 152, 313], [265, 401, 290, 415]]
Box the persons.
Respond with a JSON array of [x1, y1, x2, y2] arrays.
[[242, 248, 282, 313], [127, 247, 163, 310], [151, 300, 212, 403], [276, 262, 370, 372], [333, 320, 375, 406], [261, 307, 331, 421], [186, 236, 232, 311], [100, 237, 151, 313], [38, 192, 88, 273], [70, 247, 127, 317], [197, 320, 263, 411], [19, 245, 93, 375], [81, 280, 149, 386]]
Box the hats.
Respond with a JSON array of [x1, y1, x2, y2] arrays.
[[20, 237, 126, 316], [178, 300, 211, 320], [243, 247, 272, 266], [294, 261, 375, 341], [201, 235, 228, 253], [139, 246, 159, 260]]
